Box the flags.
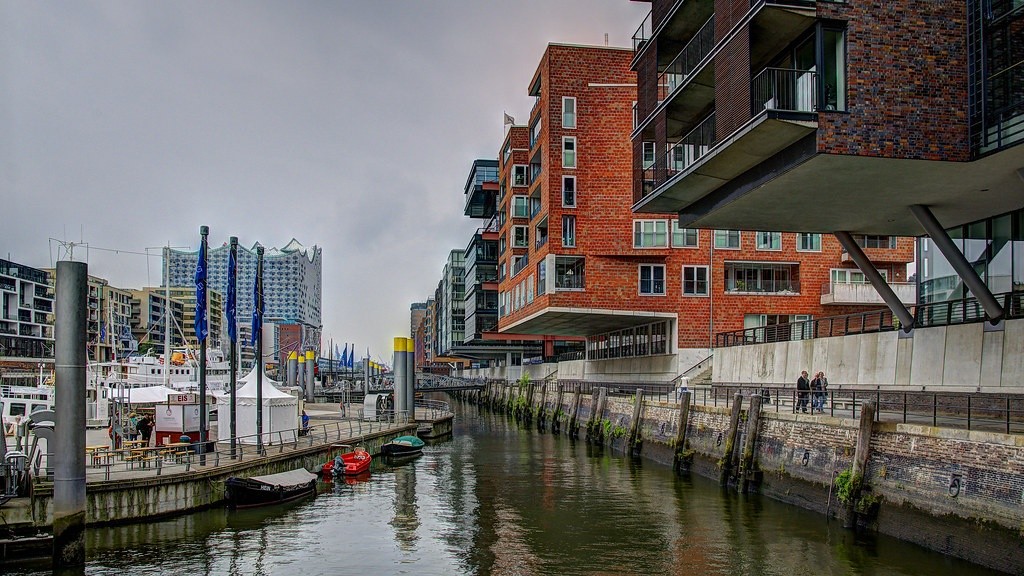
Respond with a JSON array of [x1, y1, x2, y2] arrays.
[[339, 347, 347, 367], [347, 351, 353, 368], [251, 265, 266, 345], [225, 250, 239, 344], [194, 242, 208, 341], [504, 113, 514, 125]]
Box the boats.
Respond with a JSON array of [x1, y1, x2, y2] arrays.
[[0, 244, 250, 440], [223, 468, 320, 508], [379, 434, 426, 461], [319, 443, 372, 478]]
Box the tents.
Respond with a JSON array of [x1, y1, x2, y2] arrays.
[[217, 369, 299, 445], [237, 363, 277, 382], [106, 385, 186, 404]]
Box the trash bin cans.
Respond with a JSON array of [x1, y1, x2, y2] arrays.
[[180, 434, 190, 450], [18, 424, 25, 437]]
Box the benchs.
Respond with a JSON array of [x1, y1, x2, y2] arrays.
[[86, 448, 197, 471], [826, 397, 864, 410], [769, 395, 797, 405]]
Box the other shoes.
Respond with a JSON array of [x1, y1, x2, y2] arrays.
[[802, 410, 809, 414], [816, 408, 820, 412], [820, 411, 825, 413], [796, 410, 799, 413]]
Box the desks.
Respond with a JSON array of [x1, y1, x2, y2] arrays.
[[130, 446, 169, 468], [121, 441, 148, 460], [86, 445, 110, 467], [168, 442, 193, 463]]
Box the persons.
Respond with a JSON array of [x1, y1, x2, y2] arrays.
[[317, 373, 327, 387], [116, 412, 138, 446], [811, 372, 828, 413], [795, 371, 811, 414], [678, 373, 689, 400], [302, 411, 310, 435], [137, 413, 154, 447], [8, 414, 23, 451], [339, 400, 342, 417]]
